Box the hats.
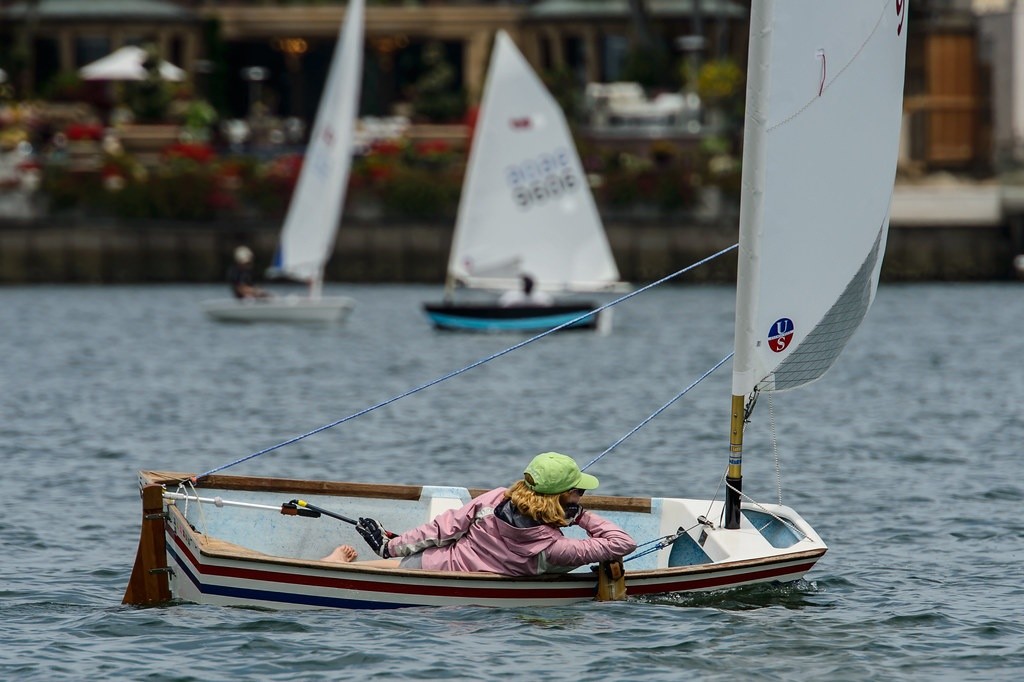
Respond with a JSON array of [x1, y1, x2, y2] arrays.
[[522, 451, 599, 495]]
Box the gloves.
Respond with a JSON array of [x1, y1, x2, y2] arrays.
[[555, 504, 584, 529], [355, 515, 388, 557]]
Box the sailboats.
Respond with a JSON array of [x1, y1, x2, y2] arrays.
[[420, 25, 637, 339], [199, 2, 363, 322], [122, 1, 912, 614]]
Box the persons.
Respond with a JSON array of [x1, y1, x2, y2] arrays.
[[226, 245, 274, 301], [322, 450, 638, 579]]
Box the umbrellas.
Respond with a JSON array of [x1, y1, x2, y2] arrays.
[[75, 43, 187, 83]]
